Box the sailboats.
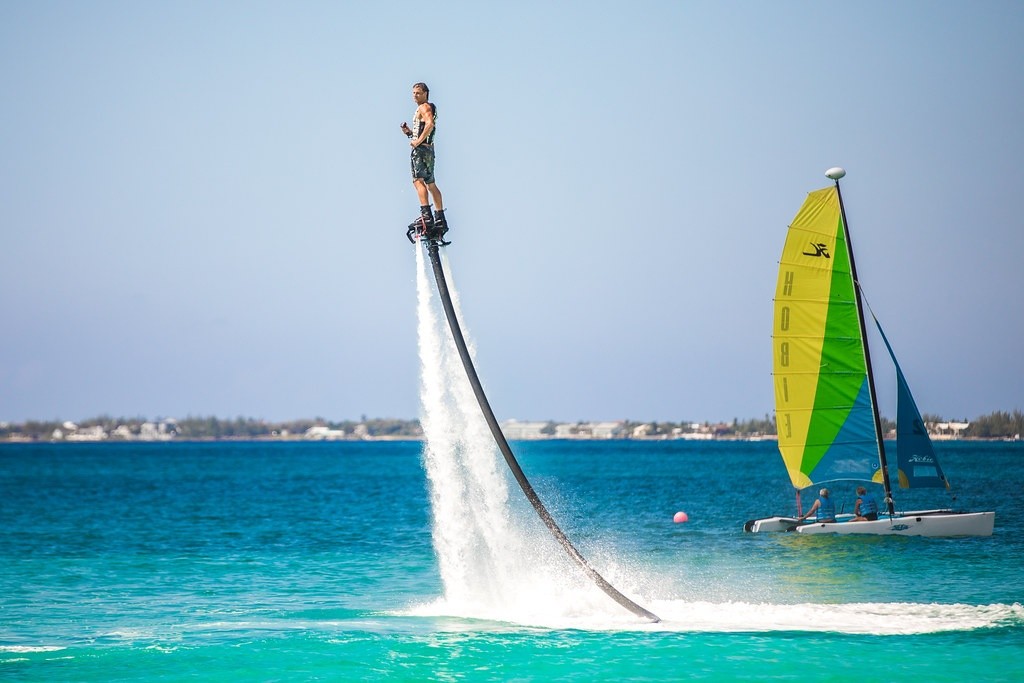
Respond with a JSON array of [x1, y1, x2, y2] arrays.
[[742, 166, 996, 536]]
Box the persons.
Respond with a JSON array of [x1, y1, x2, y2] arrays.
[[847, 487, 878, 522], [796, 487, 838, 524], [401, 82, 447, 236]]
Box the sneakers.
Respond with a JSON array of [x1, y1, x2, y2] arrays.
[[408, 214, 434, 228], [433, 219, 448, 231]]
